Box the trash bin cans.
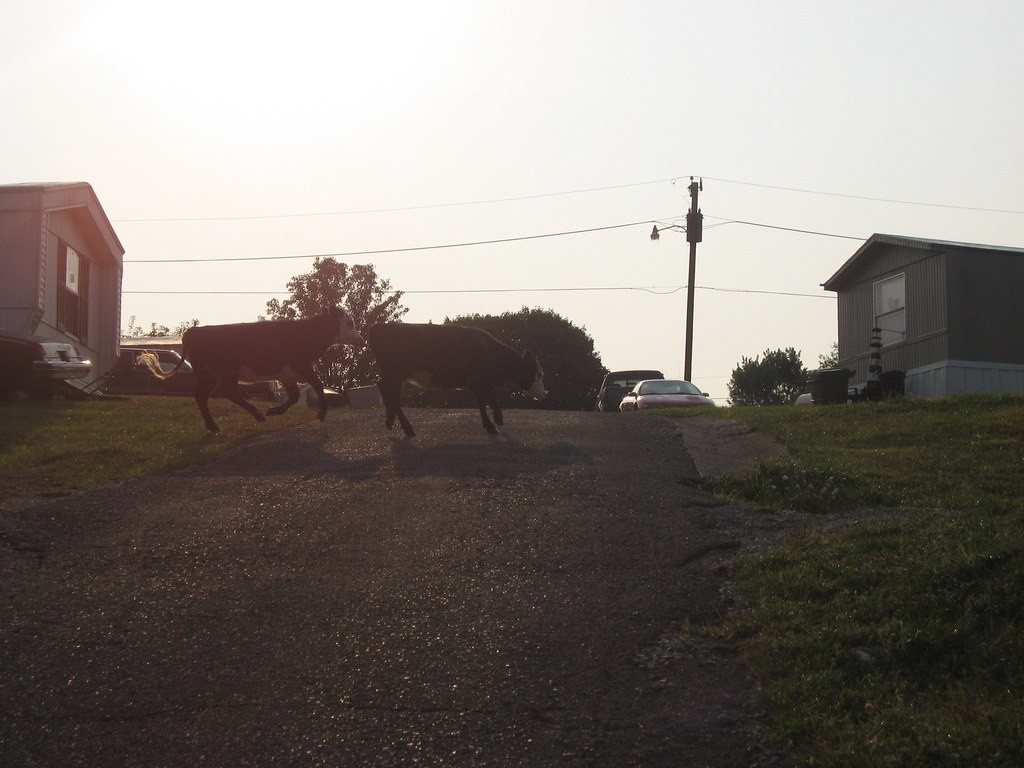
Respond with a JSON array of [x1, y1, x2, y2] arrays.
[[868, 370, 906, 402], [806, 368, 851, 405]]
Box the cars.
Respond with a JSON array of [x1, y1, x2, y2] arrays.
[[617, 378, 716, 412], [120, 347, 192, 374], [0, 327, 93, 393]]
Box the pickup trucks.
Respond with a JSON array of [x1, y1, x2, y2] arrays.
[[594, 369, 664, 412]]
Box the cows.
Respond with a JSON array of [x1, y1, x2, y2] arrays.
[[134, 304, 365, 432], [370, 321, 548, 438]]
